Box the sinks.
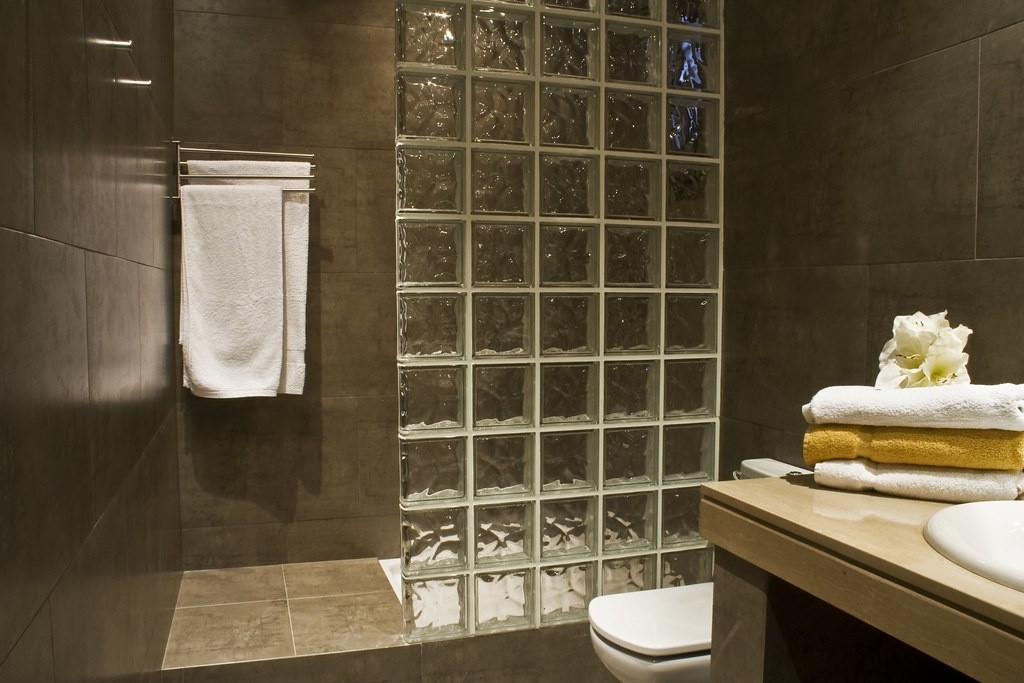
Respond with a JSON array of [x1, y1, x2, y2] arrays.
[[922, 500, 1024, 594]]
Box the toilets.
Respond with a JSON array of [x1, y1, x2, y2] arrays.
[[588, 459, 815, 683]]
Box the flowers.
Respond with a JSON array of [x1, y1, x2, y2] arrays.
[[874, 311, 976, 387]]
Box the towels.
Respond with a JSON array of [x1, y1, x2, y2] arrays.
[[0, 136, 174, 411], [178, 160, 311, 399], [801, 383, 1024, 502]]
